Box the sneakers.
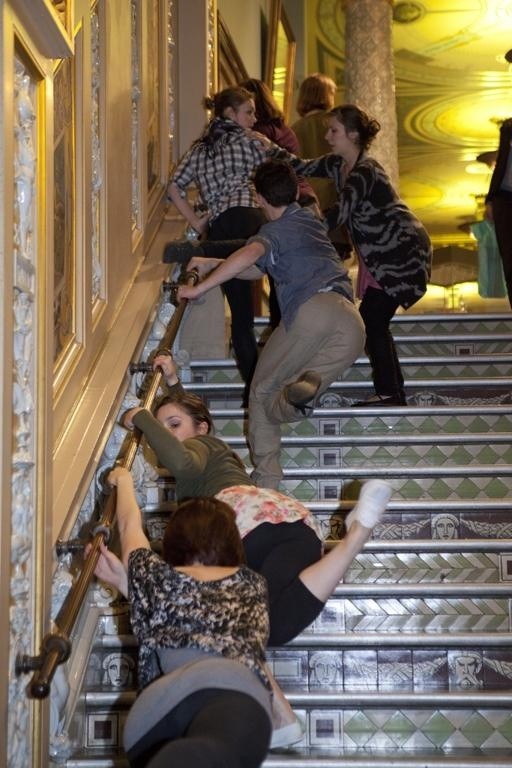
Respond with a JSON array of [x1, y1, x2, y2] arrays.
[[283, 367, 322, 405], [269, 719, 307, 751], [342, 478, 394, 535]]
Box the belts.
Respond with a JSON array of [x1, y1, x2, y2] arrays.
[[331, 284, 360, 304]]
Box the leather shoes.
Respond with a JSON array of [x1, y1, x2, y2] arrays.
[[349, 393, 404, 409]]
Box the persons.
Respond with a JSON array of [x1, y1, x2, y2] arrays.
[[238, 76, 321, 349], [76, 466, 275, 766], [246, 101, 433, 406], [484, 49, 512, 308], [289, 70, 356, 261], [175, 160, 370, 491], [168, 83, 284, 410], [120, 353, 391, 750]]
